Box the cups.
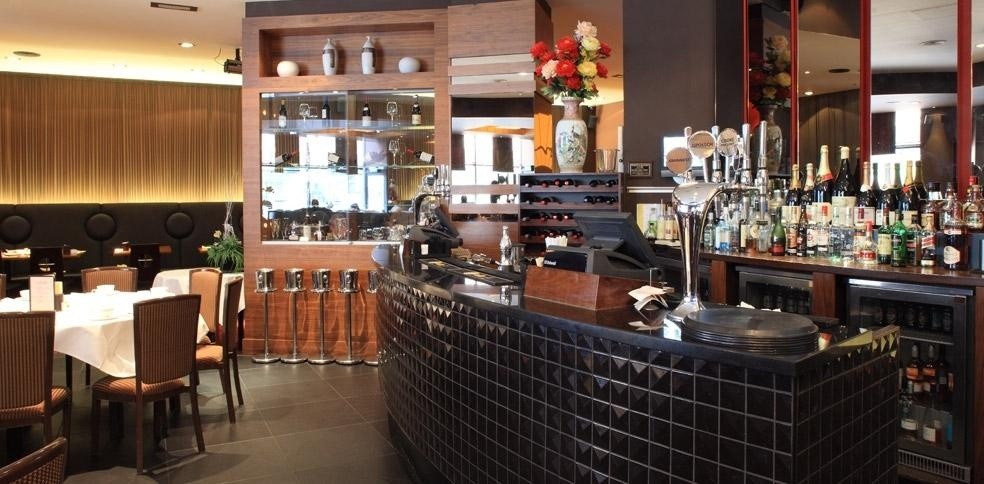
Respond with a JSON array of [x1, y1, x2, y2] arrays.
[[21, 283, 167, 322], [299, 103, 309, 119], [594, 148, 619, 173], [524, 178, 617, 239], [307, 107, 319, 119], [507, 243, 529, 266], [268, 217, 413, 241]]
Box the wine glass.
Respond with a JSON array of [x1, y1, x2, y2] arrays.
[[386, 101, 398, 128], [397, 143, 406, 165], [388, 140, 399, 166]]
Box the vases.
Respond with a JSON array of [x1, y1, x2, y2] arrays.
[[276, 59, 299, 76], [553, 95, 588, 173], [753, 105, 782, 175], [398, 56, 420, 72], [219, 256, 234, 272], [322, 35, 338, 75], [360, 34, 377, 74]]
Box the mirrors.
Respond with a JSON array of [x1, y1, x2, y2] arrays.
[[743, 0, 794, 179], [866, 0, 970, 199], [794, 0, 865, 177], [966, 0, 984, 201]]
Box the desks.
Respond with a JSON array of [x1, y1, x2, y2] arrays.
[[0, 290, 206, 448], [153, 270, 245, 344]]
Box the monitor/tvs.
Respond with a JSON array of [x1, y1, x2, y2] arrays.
[[573, 210, 664, 276], [430, 205, 459, 237]]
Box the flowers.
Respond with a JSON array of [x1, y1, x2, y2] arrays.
[[203, 229, 242, 270], [748, 34, 791, 106], [529, 17, 623, 97]]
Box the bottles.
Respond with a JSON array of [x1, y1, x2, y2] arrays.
[[362, 104, 372, 126], [279, 100, 287, 129], [645, 143, 983, 275], [404, 147, 434, 165], [322, 95, 330, 127], [270, 150, 299, 166], [411, 94, 421, 125], [499, 227, 512, 264], [761, 283, 953, 414]]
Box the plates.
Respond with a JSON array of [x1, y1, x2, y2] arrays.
[[680, 307, 820, 357]]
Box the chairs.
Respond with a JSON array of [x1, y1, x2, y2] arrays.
[[0, 305, 72, 446], [81, 265, 139, 389], [89, 292, 207, 475], [168, 267, 243, 424], [0, 435, 160, 484], [0, 272, 7, 300]]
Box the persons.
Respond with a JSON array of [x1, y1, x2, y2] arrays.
[[461, 196, 467, 204], [490, 180, 499, 203], [351, 202, 360, 212], [312, 199, 319, 207]]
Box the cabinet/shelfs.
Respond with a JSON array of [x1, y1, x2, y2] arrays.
[[517, 171, 623, 262], [653, 250, 720, 310], [259, 90, 440, 244], [825, 272, 984, 483], [720, 260, 825, 334]]
